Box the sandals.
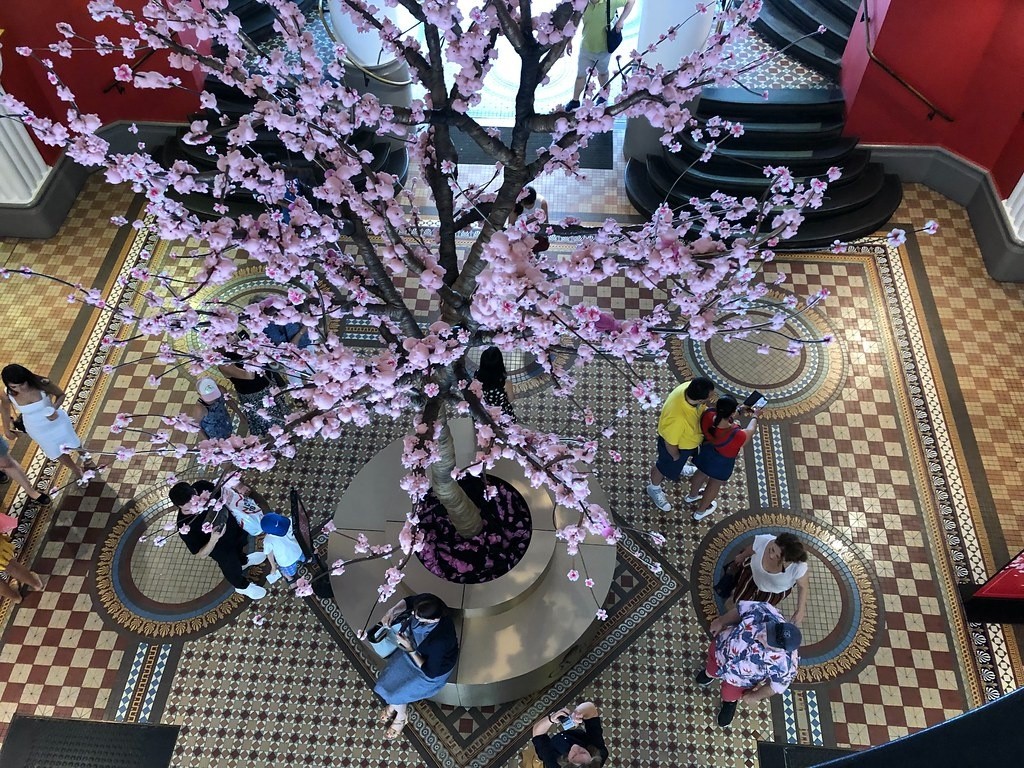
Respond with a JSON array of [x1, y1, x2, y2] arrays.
[[380, 705, 396, 724], [384, 712, 410, 739]]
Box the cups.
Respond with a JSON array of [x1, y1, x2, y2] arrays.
[[43, 406, 58, 421]]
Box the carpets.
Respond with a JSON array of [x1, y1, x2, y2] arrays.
[[448, 126, 613, 169]]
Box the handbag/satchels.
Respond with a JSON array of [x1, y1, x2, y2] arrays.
[[225, 489, 266, 537], [0, 513, 19, 534], [607, 24, 623, 54], [13, 413, 27, 434], [365, 611, 413, 659], [712, 560, 743, 599]]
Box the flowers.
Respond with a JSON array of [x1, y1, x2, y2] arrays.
[[1, 1, 939, 641]]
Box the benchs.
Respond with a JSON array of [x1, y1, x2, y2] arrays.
[[327, 418, 616, 706]]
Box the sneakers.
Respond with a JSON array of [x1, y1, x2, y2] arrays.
[[234, 582, 267, 600], [683, 488, 705, 503], [693, 500, 718, 521], [680, 463, 698, 476], [696, 667, 715, 686], [647, 484, 672, 511], [717, 700, 739, 727], [241, 551, 267, 570]]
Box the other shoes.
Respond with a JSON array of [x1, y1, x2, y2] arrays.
[[286, 577, 298, 590], [302, 556, 318, 565], [28, 492, 51, 506], [595, 97, 607, 106], [0, 470, 9, 484], [565, 99, 581, 112]]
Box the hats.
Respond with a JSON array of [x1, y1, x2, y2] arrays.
[[766, 622, 803, 653], [261, 513, 291, 537], [195, 376, 221, 403]]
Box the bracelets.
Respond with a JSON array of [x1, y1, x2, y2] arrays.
[[751, 416, 758, 423], [299, 331, 304, 335], [737, 409, 741, 415]]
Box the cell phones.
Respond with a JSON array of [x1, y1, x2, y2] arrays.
[[217, 523, 225, 533], [750, 397, 769, 412], [560, 716, 574, 730], [10, 430, 24, 438]]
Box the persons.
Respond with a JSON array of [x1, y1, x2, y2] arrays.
[[0, 390, 51, 505], [374, 594, 457, 739], [734, 532, 809, 624], [696, 600, 802, 726], [0, 364, 96, 489], [194, 294, 314, 498], [474, 347, 518, 427], [685, 395, 761, 520], [565, 0, 635, 112], [0, 532, 43, 604], [509, 187, 549, 256], [647, 377, 714, 513], [261, 513, 315, 592], [523, 702, 609, 768], [169, 480, 266, 600]]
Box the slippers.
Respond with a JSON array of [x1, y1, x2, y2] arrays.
[[75, 472, 89, 489], [80, 451, 93, 465], [30, 571, 43, 592], [9, 578, 23, 605]]
